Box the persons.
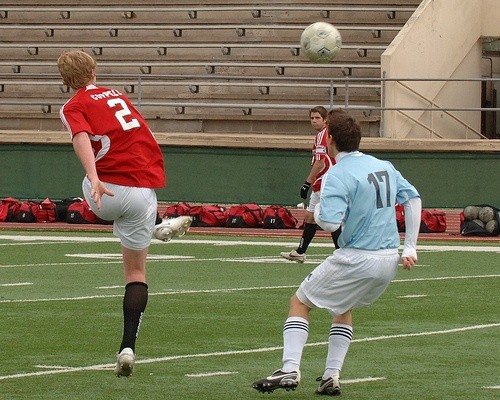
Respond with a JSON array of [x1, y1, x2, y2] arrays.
[[55, 49, 192, 379], [280, 106, 342, 264], [252, 110, 422, 394]]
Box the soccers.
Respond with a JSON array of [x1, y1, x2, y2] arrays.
[[463, 205, 500, 235], [300, 21, 343, 64]]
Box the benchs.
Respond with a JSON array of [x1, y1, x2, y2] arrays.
[[0, 0, 416, 137]]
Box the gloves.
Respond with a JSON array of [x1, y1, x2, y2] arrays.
[[300, 181, 311, 200]]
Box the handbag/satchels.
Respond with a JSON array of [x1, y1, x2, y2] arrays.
[[0, 196, 98, 224], [395, 204, 447, 232], [263, 205, 298, 228], [190, 205, 228, 226], [163, 202, 191, 219], [225, 205, 263, 226]]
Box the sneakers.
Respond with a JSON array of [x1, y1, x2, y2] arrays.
[[280, 250, 306, 264], [153, 215, 193, 242], [314, 376, 342, 396], [113, 347, 136, 377], [251, 369, 301, 394]]
